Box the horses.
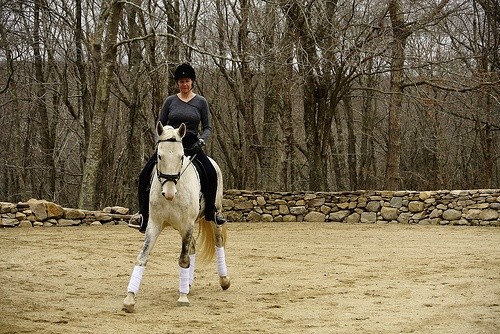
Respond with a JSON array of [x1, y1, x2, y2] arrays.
[[121, 121, 231, 312]]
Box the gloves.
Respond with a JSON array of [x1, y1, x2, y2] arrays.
[[198, 138, 205, 148]]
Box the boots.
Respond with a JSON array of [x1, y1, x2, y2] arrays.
[[137, 186, 149, 233], [203, 183, 217, 221]]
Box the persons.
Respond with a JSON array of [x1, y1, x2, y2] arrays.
[[136, 62, 227, 234]]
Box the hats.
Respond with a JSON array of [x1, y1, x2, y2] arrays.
[[175, 63, 196, 81]]
